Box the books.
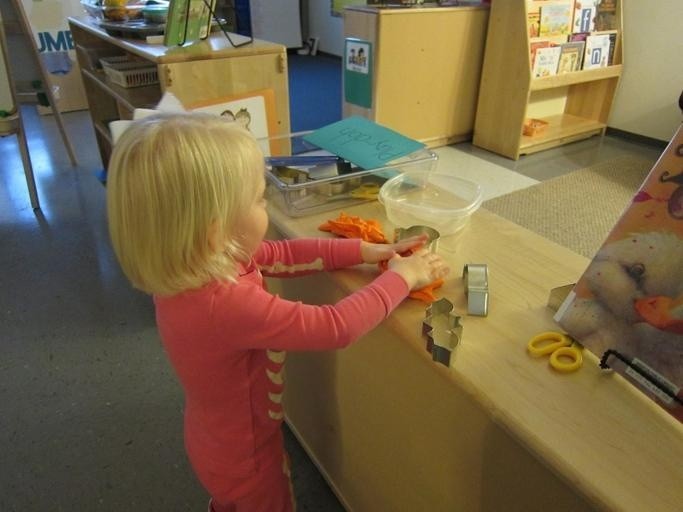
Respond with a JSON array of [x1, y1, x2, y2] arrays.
[[367, 2, 412, 9], [527, 1, 618, 80]]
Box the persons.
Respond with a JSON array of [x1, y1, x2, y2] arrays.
[[348, 48, 365, 65], [105, 112, 450, 511]]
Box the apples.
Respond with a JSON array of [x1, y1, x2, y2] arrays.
[[103, 6, 126, 20], [102, 0, 128, 6]]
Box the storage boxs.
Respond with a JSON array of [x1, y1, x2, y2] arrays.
[[260, 151, 483, 236]]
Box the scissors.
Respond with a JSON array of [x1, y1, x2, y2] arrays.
[[528, 331, 583, 373]]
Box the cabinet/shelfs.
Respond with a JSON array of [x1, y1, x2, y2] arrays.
[[472, 0, 625, 160], [66, 11, 290, 173]]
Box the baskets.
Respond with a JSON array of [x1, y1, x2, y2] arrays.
[[99, 56, 159, 88]]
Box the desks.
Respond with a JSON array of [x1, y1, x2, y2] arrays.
[[340, 5, 490, 152], [259, 184, 682, 511]]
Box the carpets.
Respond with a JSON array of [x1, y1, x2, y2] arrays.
[[481, 152, 653, 260]]
